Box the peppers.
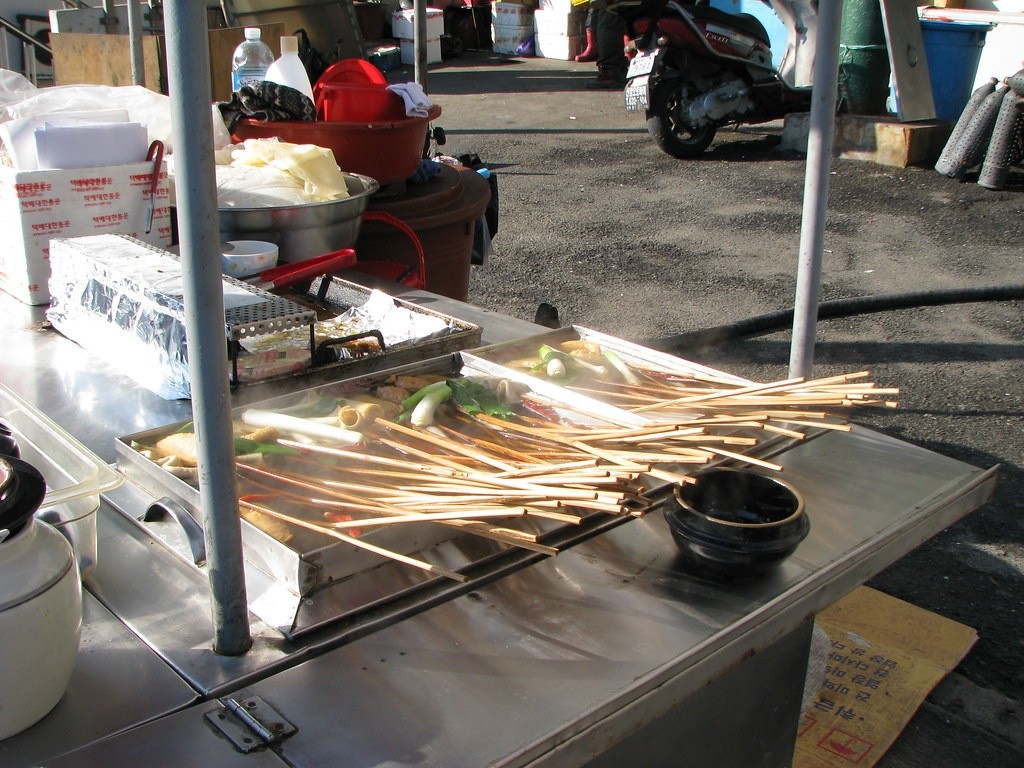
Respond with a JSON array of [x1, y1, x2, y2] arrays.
[[520, 400, 587, 429], [638, 369, 701, 383], [239, 442, 384, 539]]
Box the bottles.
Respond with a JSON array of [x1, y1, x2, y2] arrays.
[[232, 28, 274, 94], [264, 36, 315, 109]]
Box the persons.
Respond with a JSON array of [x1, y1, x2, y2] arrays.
[[575, 8, 634, 62]]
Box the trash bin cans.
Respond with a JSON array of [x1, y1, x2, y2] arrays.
[[886, 16, 997, 127], [350, 156, 492, 305]]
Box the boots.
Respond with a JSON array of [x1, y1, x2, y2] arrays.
[[624, 34, 635, 61], [575, 27, 597, 62]]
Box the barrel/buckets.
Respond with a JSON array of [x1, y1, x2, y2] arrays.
[[890, 14, 996, 118], [354, 3, 389, 38], [316, 82, 395, 121]]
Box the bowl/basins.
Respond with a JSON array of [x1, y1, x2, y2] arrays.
[[170, 171, 380, 259], [663, 467, 810, 576], [230, 104, 442, 187], [221, 241, 278, 277]]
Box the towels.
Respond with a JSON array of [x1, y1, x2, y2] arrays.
[[217, 81, 317, 136]]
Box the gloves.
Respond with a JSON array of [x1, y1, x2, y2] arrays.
[[386, 82, 433, 118]]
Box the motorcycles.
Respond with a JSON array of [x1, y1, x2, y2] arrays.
[[606, 0, 818, 160]]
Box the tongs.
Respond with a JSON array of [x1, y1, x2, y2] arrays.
[[241, 249, 357, 291]]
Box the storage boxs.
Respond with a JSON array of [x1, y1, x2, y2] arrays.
[[390, 7, 444, 65], [492, 0, 586, 60], [0, 159, 173, 307]]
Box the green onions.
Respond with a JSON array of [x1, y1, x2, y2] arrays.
[[227, 344, 648, 460]]
